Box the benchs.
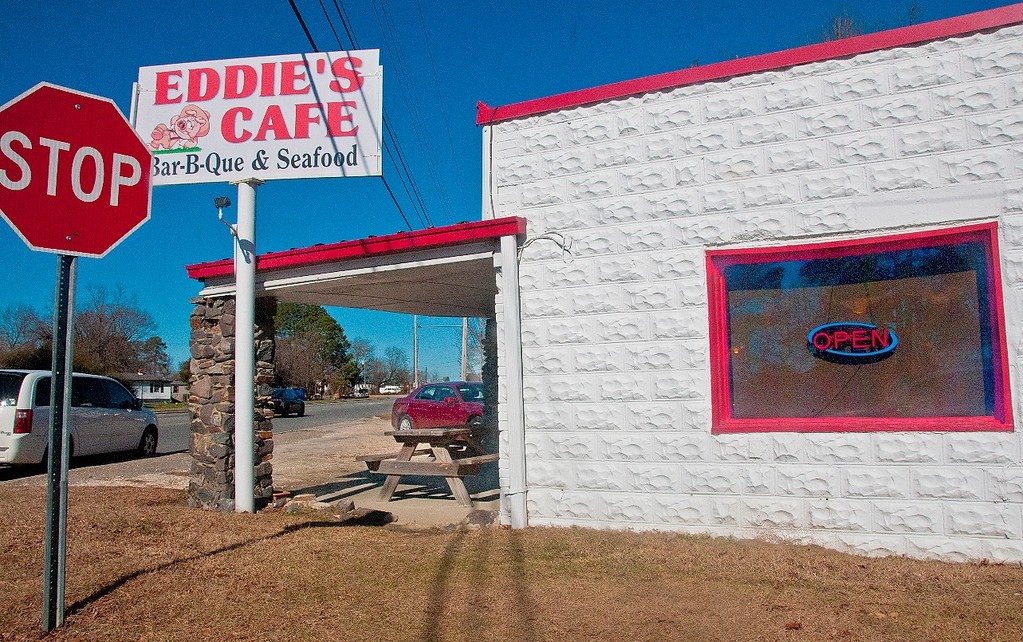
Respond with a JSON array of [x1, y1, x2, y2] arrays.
[[451, 453, 499, 467], [355, 445, 464, 461]]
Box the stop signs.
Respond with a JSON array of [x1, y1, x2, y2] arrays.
[[0, 81, 154, 257]]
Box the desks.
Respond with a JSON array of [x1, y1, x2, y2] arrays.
[[375, 425, 484, 509]]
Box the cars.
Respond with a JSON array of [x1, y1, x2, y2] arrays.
[[391, 381, 487, 432], [347, 383, 409, 398], [270, 387, 305, 418], [0, 368, 157, 468]]
